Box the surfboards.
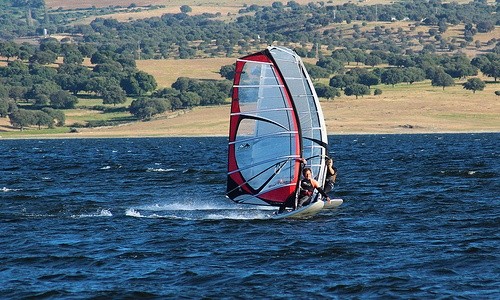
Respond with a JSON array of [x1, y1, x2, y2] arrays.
[[325, 198, 343, 207], [269, 200, 324, 219]]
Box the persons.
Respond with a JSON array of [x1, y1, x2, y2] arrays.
[[324, 159, 337, 200], [272, 168, 332, 216]]
